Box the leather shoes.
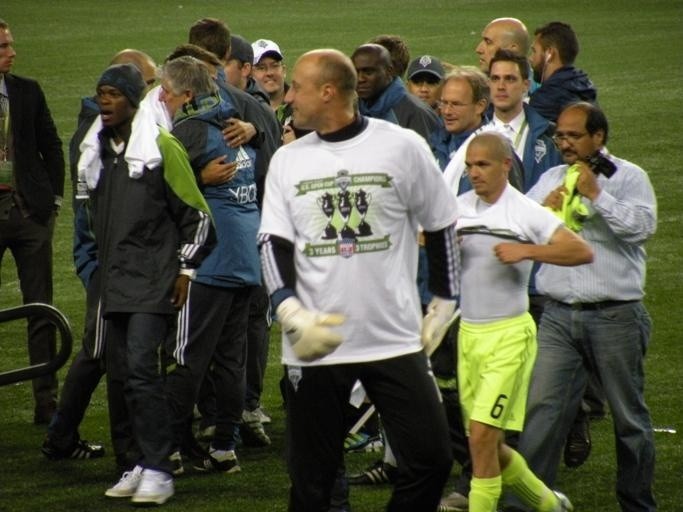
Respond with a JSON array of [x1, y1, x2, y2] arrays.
[[564, 421, 591, 467]]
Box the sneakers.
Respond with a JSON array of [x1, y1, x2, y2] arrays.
[[41, 432, 102, 460], [344, 433, 383, 454], [438, 493, 468, 512], [103, 408, 272, 505], [347, 460, 398, 486]]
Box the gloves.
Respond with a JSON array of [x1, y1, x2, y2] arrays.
[[422, 295, 457, 358], [275, 296, 345, 361]]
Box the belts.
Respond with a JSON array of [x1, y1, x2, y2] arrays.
[[552, 299, 639, 311]]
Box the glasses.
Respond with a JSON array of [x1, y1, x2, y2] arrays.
[[435, 99, 472, 111], [551, 131, 590, 145]]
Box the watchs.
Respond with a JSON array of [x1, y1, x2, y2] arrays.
[[177, 266, 196, 282]]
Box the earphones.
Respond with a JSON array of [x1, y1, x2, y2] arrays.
[[546, 53, 552, 61]]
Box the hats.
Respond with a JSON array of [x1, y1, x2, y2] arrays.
[[250, 39, 283, 65], [96, 62, 146, 108], [407, 56, 445, 80]]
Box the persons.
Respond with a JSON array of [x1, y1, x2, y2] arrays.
[[449, 130, 597, 512], [338, 18, 594, 488], [512, 100, 661, 510], [417, 63, 528, 512], [80, 61, 216, 506], [42, 19, 299, 484], [0, 20, 61, 422], [254, 47, 461, 512]]
